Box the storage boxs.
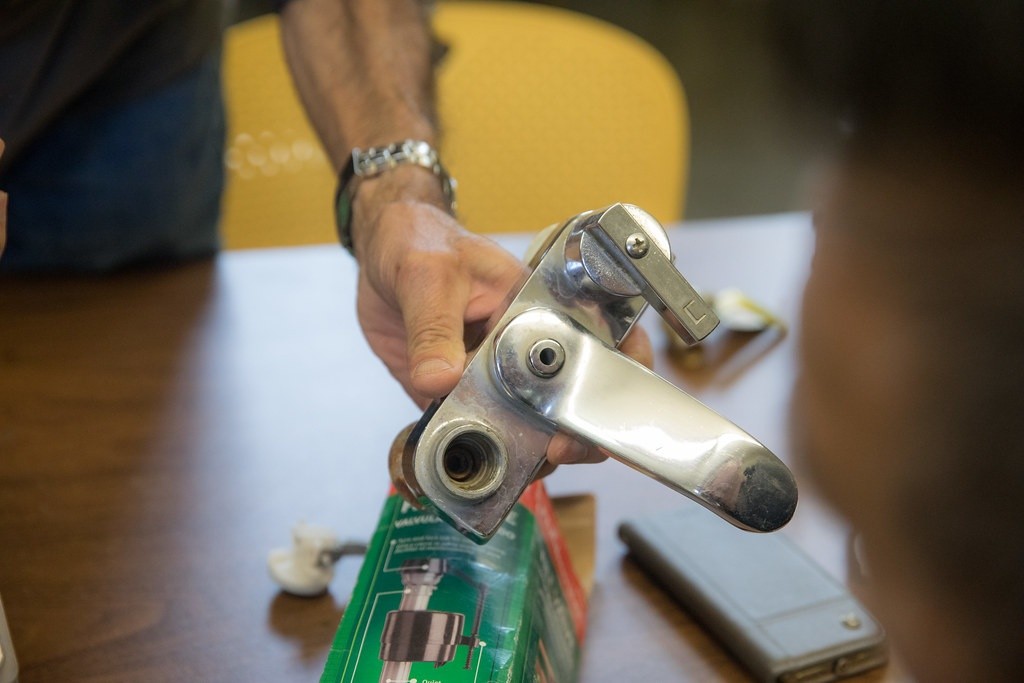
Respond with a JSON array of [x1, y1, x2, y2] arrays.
[[320, 483, 597, 683]]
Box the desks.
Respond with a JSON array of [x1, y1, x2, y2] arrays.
[[0, 208, 888, 683]]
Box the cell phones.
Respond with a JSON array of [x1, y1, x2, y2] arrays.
[[617, 506, 889, 683]]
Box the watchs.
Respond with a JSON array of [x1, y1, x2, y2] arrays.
[[334, 138, 454, 257]]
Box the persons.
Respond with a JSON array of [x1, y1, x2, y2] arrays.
[[0, 0, 654, 482]]
